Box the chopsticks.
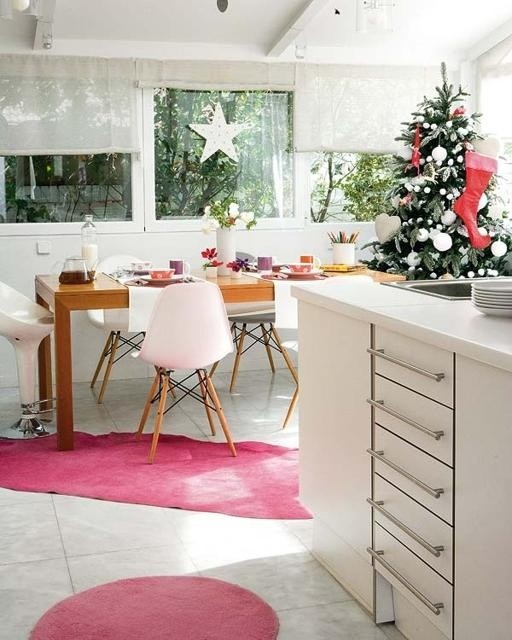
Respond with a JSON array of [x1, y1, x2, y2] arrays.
[[327, 231, 359, 243]]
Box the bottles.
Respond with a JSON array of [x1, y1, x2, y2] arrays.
[[82, 214, 98, 280]]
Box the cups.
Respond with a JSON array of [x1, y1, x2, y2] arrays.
[[115, 263, 136, 283], [169, 259, 190, 275], [253, 257, 272, 275], [300, 256, 321, 274]]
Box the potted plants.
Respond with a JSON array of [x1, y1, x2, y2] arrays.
[[203, 249, 222, 280], [202, 198, 254, 280]]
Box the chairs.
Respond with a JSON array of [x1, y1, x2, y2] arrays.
[[138, 283, 239, 463], [0, 282, 55, 438], [92, 255, 177, 404], [229, 253, 297, 394]]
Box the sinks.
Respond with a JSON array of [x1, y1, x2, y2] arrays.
[[378, 276, 504, 301]]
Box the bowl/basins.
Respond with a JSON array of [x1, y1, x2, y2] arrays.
[[130, 262, 155, 272], [288, 264, 315, 273], [149, 269, 175, 279]]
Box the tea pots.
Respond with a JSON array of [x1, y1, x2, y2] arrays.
[[50, 255, 100, 284]]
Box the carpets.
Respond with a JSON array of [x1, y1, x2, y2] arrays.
[[28, 577, 280, 640], [0, 430, 311, 519]]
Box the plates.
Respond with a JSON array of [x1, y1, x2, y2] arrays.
[[123, 269, 150, 276], [471, 280, 512, 318], [136, 275, 185, 286], [279, 269, 323, 279]]
